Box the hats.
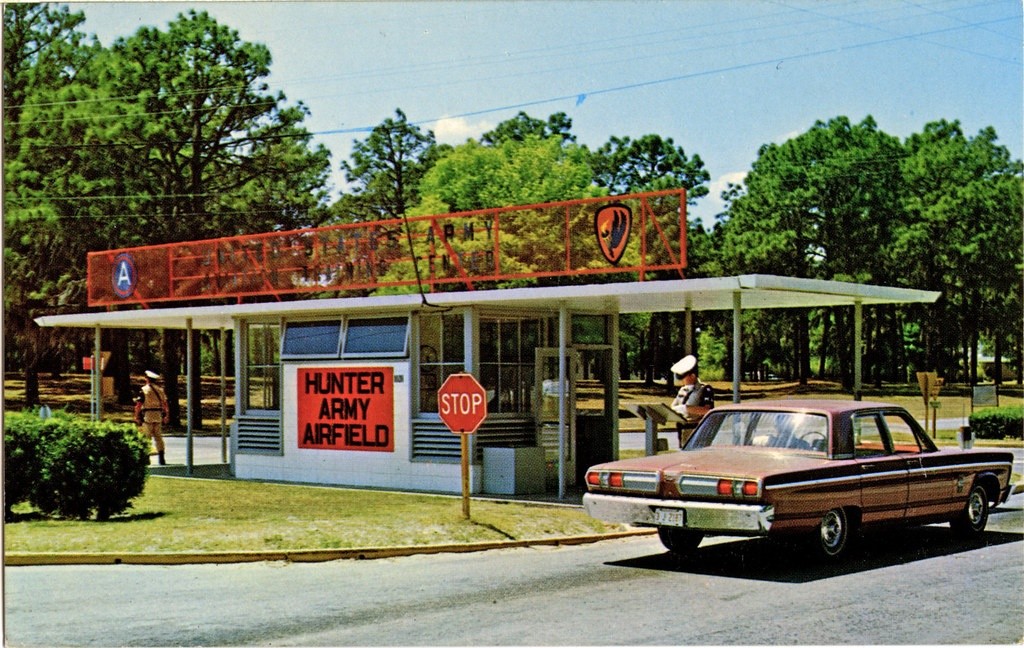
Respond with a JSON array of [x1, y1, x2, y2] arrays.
[[670, 355, 695, 380], [145, 371, 161, 381]]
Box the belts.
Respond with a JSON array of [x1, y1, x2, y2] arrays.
[[143, 407, 161, 412], [676, 423, 698, 429]]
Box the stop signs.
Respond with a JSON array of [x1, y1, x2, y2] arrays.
[[437, 374, 488, 434]]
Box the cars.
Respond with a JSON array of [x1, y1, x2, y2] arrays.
[[582, 399, 1017, 568]]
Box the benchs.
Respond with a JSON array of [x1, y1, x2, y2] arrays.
[[856, 447, 885, 457]]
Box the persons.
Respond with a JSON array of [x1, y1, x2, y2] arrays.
[[670, 354, 714, 450], [134, 370, 170, 465], [767, 415, 812, 451]]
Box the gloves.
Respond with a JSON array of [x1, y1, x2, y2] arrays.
[[673, 405, 687, 414]]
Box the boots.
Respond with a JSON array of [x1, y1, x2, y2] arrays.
[[158, 451, 166, 465]]
